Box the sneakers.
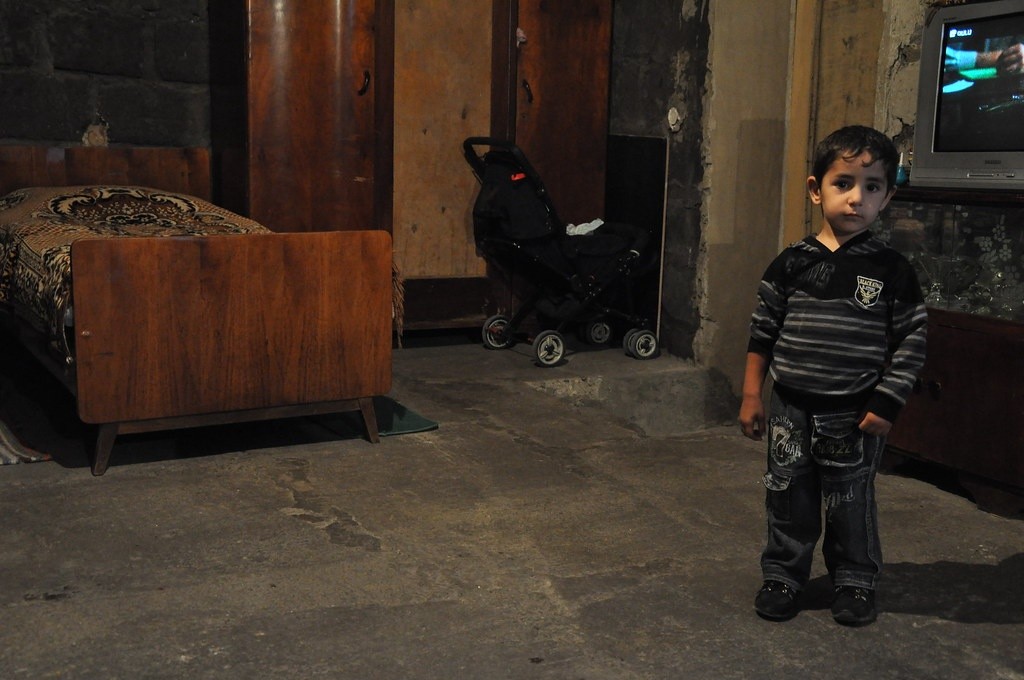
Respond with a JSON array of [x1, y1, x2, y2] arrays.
[[752, 580, 799, 619], [831, 586, 877, 624]]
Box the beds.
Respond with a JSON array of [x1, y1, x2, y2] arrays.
[[0, 138, 396, 477]]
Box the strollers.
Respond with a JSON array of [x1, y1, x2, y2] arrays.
[[461, 134, 660, 368]]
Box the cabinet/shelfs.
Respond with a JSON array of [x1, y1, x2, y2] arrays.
[[867, 189, 1024, 490]]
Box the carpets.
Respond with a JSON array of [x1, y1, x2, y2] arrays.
[[0, 420, 54, 467], [305, 395, 439, 441]]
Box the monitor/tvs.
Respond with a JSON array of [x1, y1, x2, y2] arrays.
[[909, 0, 1024, 193]]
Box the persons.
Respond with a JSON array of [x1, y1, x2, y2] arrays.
[[740, 125, 928, 623], [944, 44, 1024, 75]]
